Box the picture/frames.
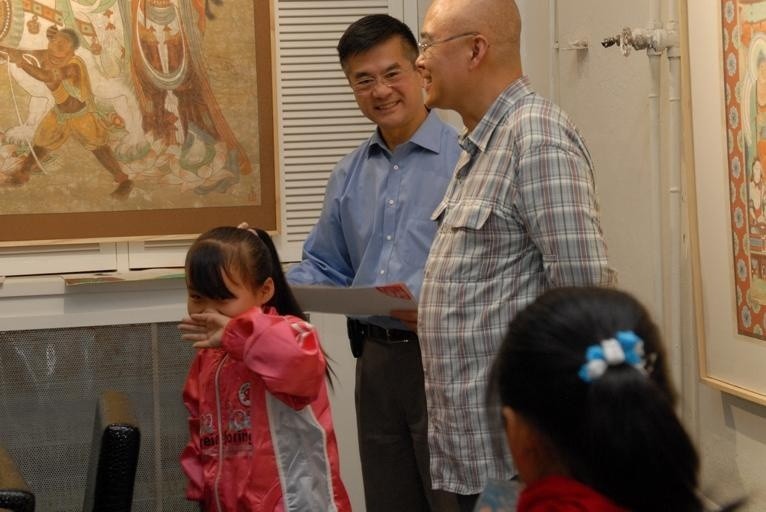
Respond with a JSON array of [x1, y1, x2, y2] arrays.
[[674, 1, 766, 410], [0, 0, 286, 248]]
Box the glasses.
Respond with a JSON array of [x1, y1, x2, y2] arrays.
[[352, 69, 405, 96], [416, 32, 477, 55]]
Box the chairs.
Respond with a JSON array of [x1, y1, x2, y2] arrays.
[[0, 392, 147, 512]]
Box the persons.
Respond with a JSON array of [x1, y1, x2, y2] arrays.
[[742, 48, 765, 237], [278, 13, 480, 512], [117, 1, 256, 177], [476, 282, 711, 512], [172, 221, 355, 512], [0, 27, 138, 205], [409, 0, 617, 511]]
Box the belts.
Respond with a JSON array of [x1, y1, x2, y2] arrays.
[[364, 324, 415, 346]]
